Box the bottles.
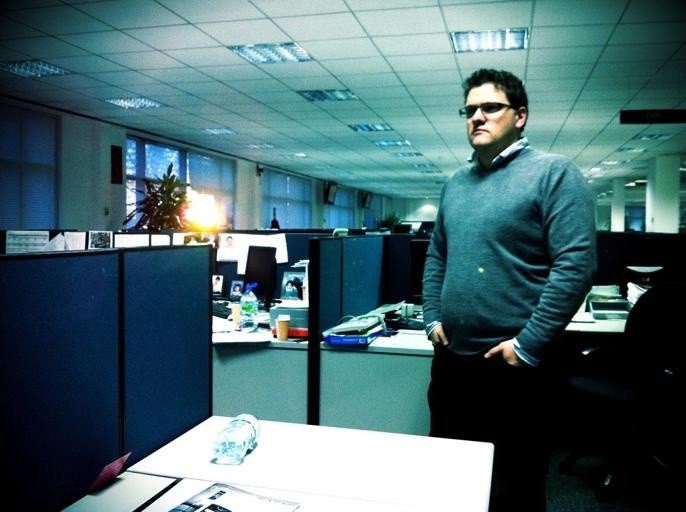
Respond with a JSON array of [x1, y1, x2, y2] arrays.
[[209, 412, 259, 466], [238, 283, 259, 332]]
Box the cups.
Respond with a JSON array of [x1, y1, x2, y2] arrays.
[[275, 318, 290, 341]]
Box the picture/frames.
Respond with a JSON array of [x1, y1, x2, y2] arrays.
[[89, 231, 113, 250], [212, 273, 245, 301], [278, 265, 306, 300]]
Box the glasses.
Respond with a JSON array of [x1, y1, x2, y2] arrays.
[[459, 103, 518, 118]]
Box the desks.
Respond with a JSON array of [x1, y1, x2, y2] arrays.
[[558, 311, 625, 436], [212, 310, 435, 436], [50, 412, 496, 512]]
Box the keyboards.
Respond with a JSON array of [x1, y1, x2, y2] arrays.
[[212, 303, 232, 319]]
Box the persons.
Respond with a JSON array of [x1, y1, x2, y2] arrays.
[[230, 285, 242, 296], [421, 68, 599, 511], [226, 236, 233, 247], [286, 281, 299, 297], [212, 277, 221, 293]]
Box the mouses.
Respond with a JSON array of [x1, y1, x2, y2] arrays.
[[228, 313, 248, 321]]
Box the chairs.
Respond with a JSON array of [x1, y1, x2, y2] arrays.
[[559, 271, 686, 501]]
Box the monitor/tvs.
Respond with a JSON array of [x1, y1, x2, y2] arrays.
[[363, 192, 373, 210], [327, 181, 338, 205], [242, 246, 276, 311], [380, 239, 430, 330]]
